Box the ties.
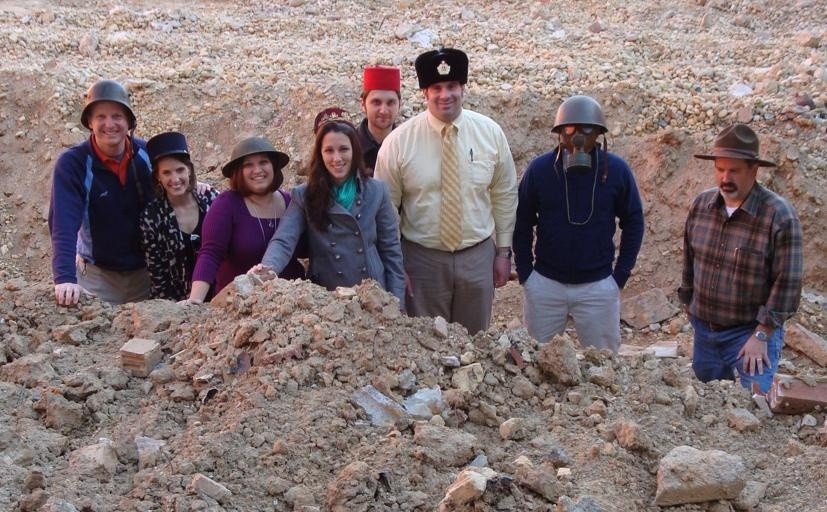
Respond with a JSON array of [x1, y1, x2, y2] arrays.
[[441, 125, 461, 252]]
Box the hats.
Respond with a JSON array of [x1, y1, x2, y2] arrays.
[[694, 123, 776, 167], [314, 49, 468, 135], [223, 137, 289, 178], [146, 132, 189, 163]]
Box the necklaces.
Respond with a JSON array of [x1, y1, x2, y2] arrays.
[[249, 195, 277, 249], [562, 143, 599, 225]]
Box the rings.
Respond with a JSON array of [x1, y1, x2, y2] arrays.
[[756, 358, 763, 362]]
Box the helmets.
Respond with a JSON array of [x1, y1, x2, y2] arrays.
[[81, 80, 137, 130], [551, 95, 608, 133]]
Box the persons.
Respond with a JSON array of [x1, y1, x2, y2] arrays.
[[176, 136, 311, 308], [677, 124, 803, 394], [372, 48, 519, 336], [355, 65, 403, 177], [139, 132, 222, 303], [513, 95, 644, 357], [261, 107, 406, 317], [48, 81, 151, 306]]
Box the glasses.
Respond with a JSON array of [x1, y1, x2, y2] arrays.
[[190, 233, 201, 262]]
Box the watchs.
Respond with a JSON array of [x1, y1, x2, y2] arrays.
[[497, 250, 514, 259], [754, 328, 771, 345]]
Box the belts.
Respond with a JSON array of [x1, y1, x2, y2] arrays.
[[709, 320, 722, 331], [454, 236, 493, 252]]
